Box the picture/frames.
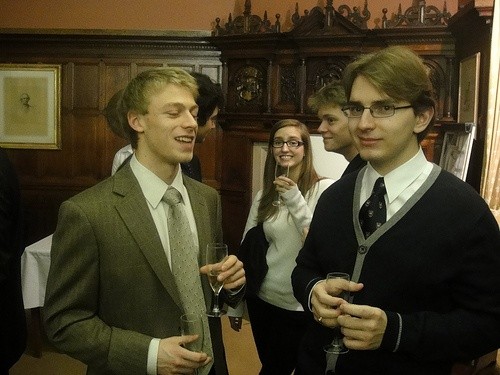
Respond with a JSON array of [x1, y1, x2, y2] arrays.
[[438, 131, 474, 182], [0, 64, 62, 150], [457, 51, 480, 122]]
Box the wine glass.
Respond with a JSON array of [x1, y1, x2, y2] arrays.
[[272, 158, 290, 207], [323, 272, 351, 354], [180, 313, 204, 375], [205, 243, 228, 317]]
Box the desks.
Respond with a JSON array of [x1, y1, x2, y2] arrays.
[[20, 230, 53, 359]]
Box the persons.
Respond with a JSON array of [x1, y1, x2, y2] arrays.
[[41, 67, 246, 375], [307, 79, 368, 181], [116, 71, 224, 184], [291, 46, 500, 375], [226, 120, 340, 375]]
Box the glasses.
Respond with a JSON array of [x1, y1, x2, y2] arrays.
[[272, 140, 305, 149], [342, 102, 413, 119]]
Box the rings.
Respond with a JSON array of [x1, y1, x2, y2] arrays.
[[318, 317, 322, 322]]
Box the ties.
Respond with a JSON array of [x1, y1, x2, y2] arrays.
[[358, 177, 387, 240], [160, 188, 215, 374]]
